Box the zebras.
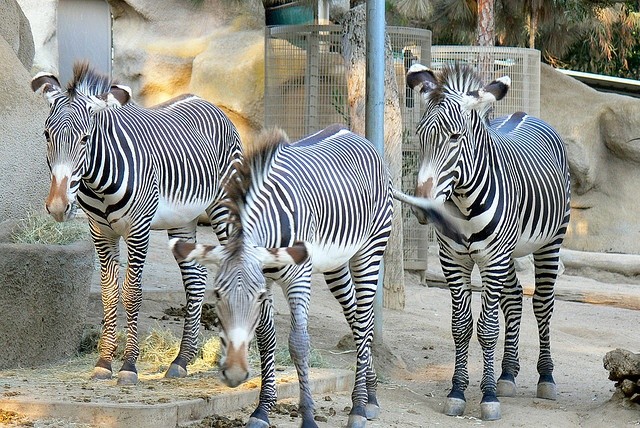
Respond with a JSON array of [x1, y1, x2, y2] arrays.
[[404, 60, 573, 421], [30, 59, 246, 390], [167, 123, 463, 427]]
